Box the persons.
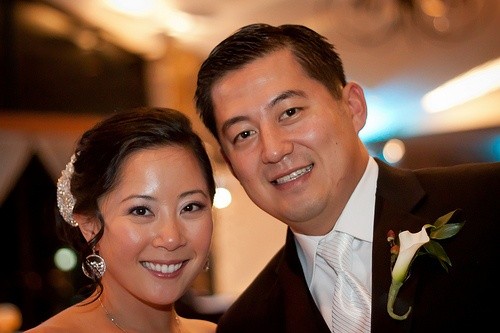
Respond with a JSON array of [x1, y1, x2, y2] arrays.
[[21, 107, 218, 333], [194, 22, 500, 333]]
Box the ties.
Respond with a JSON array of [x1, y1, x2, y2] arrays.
[[315, 232, 372, 333]]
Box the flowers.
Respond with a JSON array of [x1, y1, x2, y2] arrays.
[[386, 208, 467, 321]]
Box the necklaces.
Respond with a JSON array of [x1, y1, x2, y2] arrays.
[[97, 289, 181, 333]]
